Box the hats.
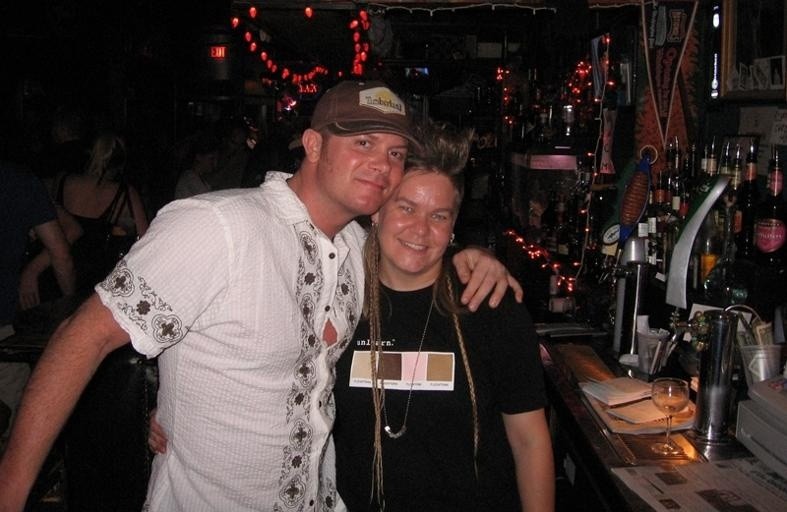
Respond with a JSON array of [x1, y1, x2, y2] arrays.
[[311, 79, 422, 151]]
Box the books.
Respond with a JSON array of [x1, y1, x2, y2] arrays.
[[578, 374, 665, 406], [576, 380, 696, 436], [605, 397, 688, 424]]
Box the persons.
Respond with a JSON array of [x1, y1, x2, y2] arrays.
[[0, 74, 525, 512], [0, 105, 309, 512], [146, 118, 556, 510]]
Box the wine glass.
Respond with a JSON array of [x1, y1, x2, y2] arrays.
[[648, 377, 691, 457]]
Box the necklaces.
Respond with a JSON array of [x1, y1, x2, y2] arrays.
[[370, 278, 434, 439]]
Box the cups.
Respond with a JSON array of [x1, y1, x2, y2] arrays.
[[635, 326, 671, 376], [738, 343, 781, 390]]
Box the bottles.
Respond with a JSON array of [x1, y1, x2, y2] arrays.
[[635, 134, 787, 308]]
